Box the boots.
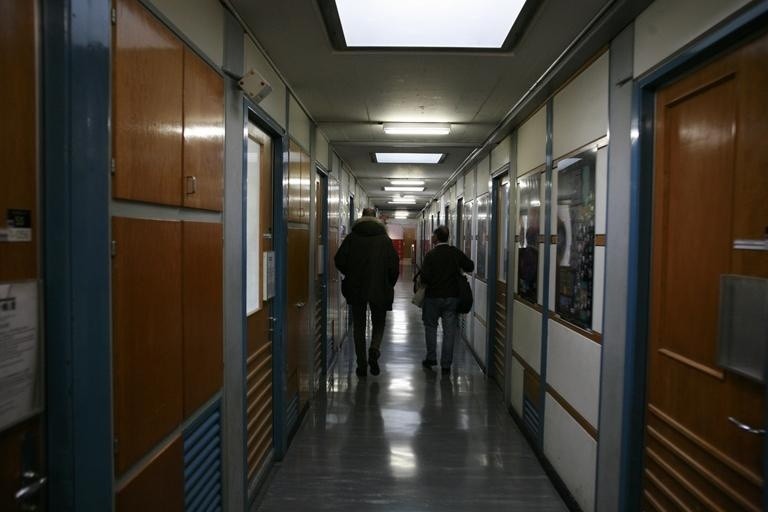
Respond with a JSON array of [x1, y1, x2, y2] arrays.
[[368, 348, 381, 376]]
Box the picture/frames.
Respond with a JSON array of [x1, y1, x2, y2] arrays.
[[264, 249, 276, 302], [0, 279, 45, 433]]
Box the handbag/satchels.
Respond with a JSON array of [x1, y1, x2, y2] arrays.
[[457, 277, 473, 313], [412, 287, 425, 307]]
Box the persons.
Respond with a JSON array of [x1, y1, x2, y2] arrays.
[[410, 226, 476, 379], [334, 206, 401, 379], [516, 227, 537, 302]]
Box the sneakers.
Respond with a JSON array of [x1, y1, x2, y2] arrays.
[[442, 368, 450, 376], [356, 360, 368, 379], [422, 360, 437, 367]]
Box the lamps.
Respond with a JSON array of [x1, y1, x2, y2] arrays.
[[383, 122, 451, 135]]
